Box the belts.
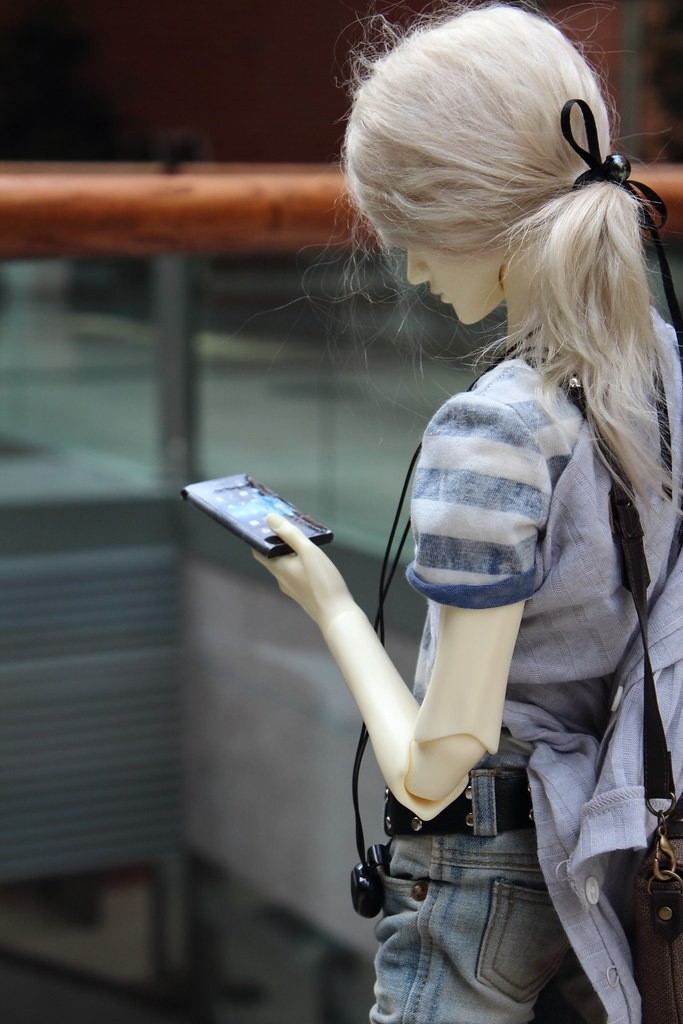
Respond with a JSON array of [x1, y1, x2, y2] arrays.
[[384, 766, 534, 836]]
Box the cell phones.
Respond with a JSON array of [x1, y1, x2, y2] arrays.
[[182, 474, 334, 559]]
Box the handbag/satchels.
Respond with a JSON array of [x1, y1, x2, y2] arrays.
[[630, 792, 683, 1024]]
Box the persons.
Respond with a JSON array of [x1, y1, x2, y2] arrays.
[[252, 0, 682, 1024]]
